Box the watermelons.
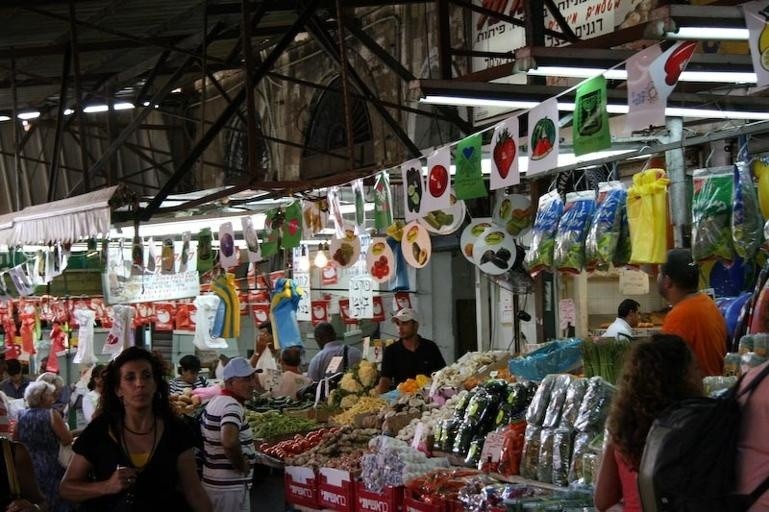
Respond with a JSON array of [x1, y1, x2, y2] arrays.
[[528, 114, 557, 161]]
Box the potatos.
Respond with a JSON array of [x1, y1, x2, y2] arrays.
[[169, 387, 201, 414]]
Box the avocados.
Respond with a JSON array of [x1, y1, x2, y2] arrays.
[[419, 249, 428, 266], [411, 241, 421, 262]]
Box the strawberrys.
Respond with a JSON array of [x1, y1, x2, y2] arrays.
[[492, 126, 518, 181]]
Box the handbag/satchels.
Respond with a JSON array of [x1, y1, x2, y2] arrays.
[[180, 402, 209, 479], [50, 408, 75, 470], [297, 373, 343, 402], [78, 414, 129, 512], [68, 395, 86, 431]]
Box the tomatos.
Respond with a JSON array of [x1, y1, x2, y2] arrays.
[[261, 428, 337, 461]]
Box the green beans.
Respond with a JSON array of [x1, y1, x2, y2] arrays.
[[252, 415, 320, 437]]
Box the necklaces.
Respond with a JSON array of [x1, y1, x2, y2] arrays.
[[121, 421, 157, 469]]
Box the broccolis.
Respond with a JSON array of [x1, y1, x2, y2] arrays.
[[327, 359, 377, 408]]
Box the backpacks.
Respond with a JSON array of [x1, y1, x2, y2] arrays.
[[639, 394, 741, 511]]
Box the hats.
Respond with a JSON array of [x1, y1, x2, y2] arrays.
[[223, 357, 262, 381], [7, 360, 20, 375], [391, 307, 419, 324], [180, 356, 202, 372], [662, 248, 693, 265]]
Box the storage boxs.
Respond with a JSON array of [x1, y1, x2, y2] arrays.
[[283, 464, 315, 510], [316, 471, 356, 512], [510, 338, 588, 382], [354, 475, 405, 512]]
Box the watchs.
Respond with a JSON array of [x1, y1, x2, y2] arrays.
[[253, 352, 261, 357]]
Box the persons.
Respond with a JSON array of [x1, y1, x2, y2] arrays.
[[58, 346, 211, 512], [601, 298, 641, 340], [307, 321, 363, 379], [735, 355, 769, 512], [34, 370, 64, 402], [247, 322, 278, 390], [168, 354, 213, 419], [12, 380, 72, 502], [596, 333, 703, 512], [200, 357, 264, 512], [272, 347, 311, 397], [706, 252, 749, 298], [1, 359, 32, 432], [654, 247, 728, 379], [369, 308, 450, 392], [80, 364, 107, 422]]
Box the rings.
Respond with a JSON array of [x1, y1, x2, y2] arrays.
[[127, 479, 132, 484]]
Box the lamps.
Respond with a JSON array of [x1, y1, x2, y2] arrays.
[[412, 79, 768, 121], [518, 48, 761, 83], [315, 243, 328, 269]]
